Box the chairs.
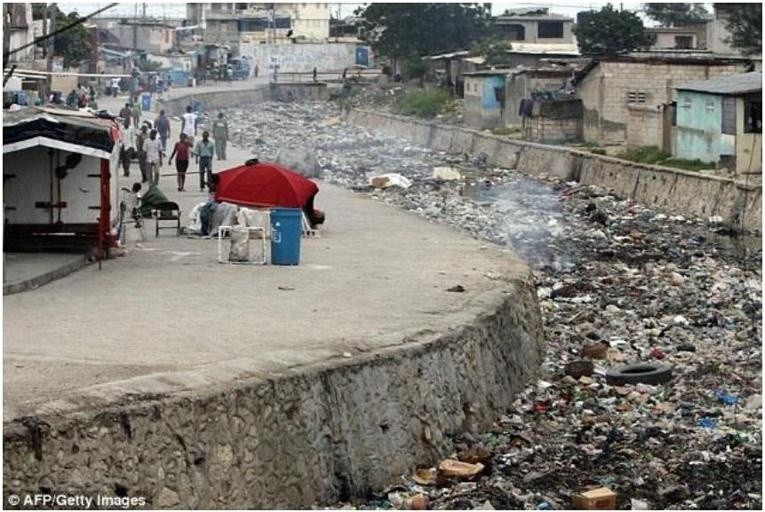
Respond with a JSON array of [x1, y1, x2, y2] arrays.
[[153, 201, 182, 237]]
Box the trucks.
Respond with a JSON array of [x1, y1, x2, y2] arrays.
[[220, 58, 249, 78]]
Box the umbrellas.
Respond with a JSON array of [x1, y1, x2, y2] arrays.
[[215, 160, 319, 210]]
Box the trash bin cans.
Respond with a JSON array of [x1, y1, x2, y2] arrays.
[[142, 93, 152, 111], [270, 209, 302, 265]]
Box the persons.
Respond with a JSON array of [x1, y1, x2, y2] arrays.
[[342, 68, 348, 83], [312, 66, 317, 82], [35, 54, 260, 227]]
